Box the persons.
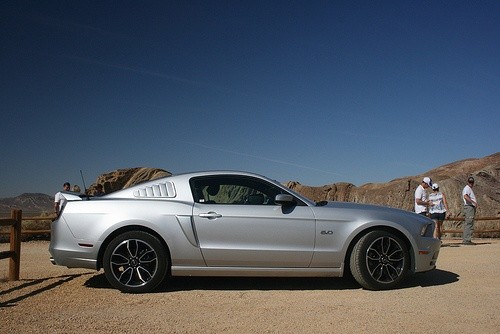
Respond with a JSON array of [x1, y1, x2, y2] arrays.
[[73, 184, 81, 193], [54, 181, 71, 217], [428, 183, 449, 242], [415, 177, 432, 216], [95, 183, 104, 195], [462, 177, 478, 245]]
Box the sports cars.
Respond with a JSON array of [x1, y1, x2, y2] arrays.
[[47, 169, 441, 294]]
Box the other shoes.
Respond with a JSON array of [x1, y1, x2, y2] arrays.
[[463, 241, 475, 245]]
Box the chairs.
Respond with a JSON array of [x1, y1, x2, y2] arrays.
[[205, 182, 220, 204]]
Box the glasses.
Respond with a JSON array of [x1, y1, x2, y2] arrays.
[[468, 180, 474, 183]]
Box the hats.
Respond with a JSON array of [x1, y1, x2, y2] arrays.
[[431, 183, 440, 189], [422, 176, 432, 187]]
